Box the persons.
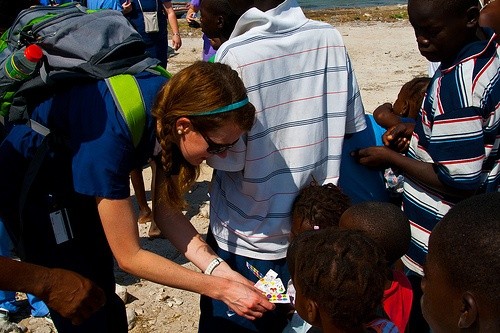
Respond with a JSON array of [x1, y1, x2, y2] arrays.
[[0, 61, 274, 333], [286, 0, 500, 333], [122, 0, 236, 74], [198, 0, 366, 333], [0, 221, 106, 326]]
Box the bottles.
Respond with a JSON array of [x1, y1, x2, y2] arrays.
[[13, 44, 43, 75]]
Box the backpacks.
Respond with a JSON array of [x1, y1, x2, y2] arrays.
[[0, 5, 174, 151]]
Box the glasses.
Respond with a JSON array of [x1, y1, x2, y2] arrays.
[[197, 123, 241, 156]]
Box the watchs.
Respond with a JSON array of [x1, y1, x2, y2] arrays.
[[172, 33, 180, 36]]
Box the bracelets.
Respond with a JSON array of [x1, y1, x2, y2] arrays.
[[205, 258, 224, 275]]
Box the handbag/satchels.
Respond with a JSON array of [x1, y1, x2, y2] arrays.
[[142, 11, 160, 32]]
